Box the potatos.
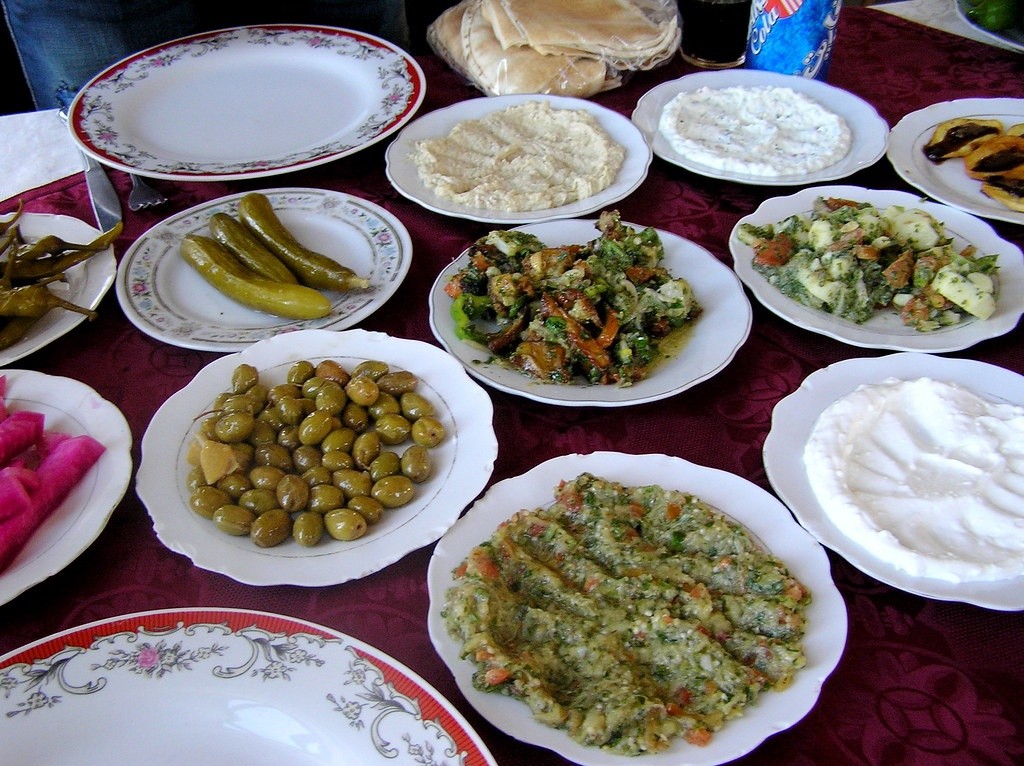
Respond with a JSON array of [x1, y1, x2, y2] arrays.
[[794, 207, 997, 320]]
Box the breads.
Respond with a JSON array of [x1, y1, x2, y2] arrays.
[[926, 115, 1024, 213]]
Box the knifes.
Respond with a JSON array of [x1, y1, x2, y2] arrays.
[[58, 105, 122, 233]]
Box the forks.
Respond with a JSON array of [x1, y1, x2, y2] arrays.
[[129, 173, 168, 214]]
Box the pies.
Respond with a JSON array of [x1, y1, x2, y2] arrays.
[[436, 1, 686, 98]]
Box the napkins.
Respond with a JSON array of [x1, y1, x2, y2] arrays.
[[0, 109, 84, 203]]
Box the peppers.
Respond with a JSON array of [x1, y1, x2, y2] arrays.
[[0, 198, 123, 348], [454, 294, 621, 369]]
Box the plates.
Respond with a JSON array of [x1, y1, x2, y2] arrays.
[[115, 188, 413, 353], [385, 93, 654, 225], [763, 353, 1024, 610], [631, 69, 889, 186], [136, 329, 498, 587], [1, 607, 499, 765], [427, 451, 848, 765], [0, 370, 132, 613], [0, 211, 117, 367], [956, 1, 1024, 52], [67, 24, 427, 182], [729, 185, 1024, 353], [887, 98, 1024, 225], [428, 218, 753, 408]]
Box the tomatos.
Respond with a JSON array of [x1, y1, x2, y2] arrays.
[[753, 232, 792, 266]]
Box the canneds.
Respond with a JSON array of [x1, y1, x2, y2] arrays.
[[746, 0, 844, 83]]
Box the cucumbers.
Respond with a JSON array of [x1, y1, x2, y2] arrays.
[[181, 191, 371, 320]]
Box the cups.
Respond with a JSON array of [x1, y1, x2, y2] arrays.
[[679, 0, 752, 67]]
